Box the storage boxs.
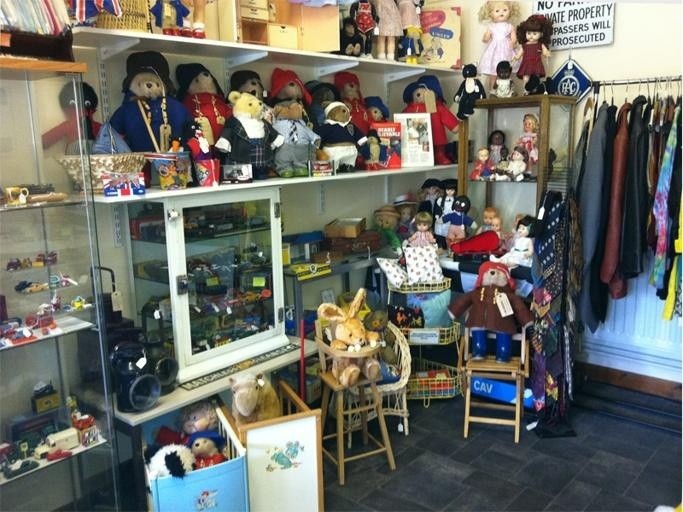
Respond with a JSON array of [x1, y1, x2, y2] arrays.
[[240, 1, 300, 51], [324, 231, 380, 256], [217, 381, 327, 512], [142, 400, 253, 510]]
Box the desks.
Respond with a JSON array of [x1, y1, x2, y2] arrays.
[[73, 333, 320, 512]]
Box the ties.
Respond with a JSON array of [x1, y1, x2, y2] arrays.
[[532, 196, 582, 416]]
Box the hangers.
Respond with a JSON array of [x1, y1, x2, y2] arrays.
[[582, 75, 682, 117]]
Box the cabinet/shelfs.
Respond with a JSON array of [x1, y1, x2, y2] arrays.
[[386, 272, 465, 405], [281, 251, 384, 407], [126, 186, 288, 385], [63, 23, 468, 210], [2, 56, 121, 510], [457, 94, 576, 237]]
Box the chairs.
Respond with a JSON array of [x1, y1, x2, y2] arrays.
[[454, 305, 533, 444], [313, 313, 413, 450]]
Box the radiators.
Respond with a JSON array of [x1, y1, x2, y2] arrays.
[[574, 246, 681, 372]]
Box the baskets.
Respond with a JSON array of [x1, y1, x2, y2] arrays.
[[95, 0, 149, 31]]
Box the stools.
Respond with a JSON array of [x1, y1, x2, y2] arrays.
[[319, 369, 397, 487]]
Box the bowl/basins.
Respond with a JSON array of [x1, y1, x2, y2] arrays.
[[52, 153, 146, 195]]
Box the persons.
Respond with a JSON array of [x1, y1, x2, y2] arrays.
[[477, 2, 522, 90], [371, 177, 539, 270], [470, 113, 541, 182], [179, 0, 207, 38], [490, 61, 518, 98], [514, 14, 554, 94], [349, 0, 425, 63]]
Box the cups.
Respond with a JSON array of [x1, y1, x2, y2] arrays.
[[191, 160, 222, 186], [152, 151, 189, 190], [3, 186, 29, 207]]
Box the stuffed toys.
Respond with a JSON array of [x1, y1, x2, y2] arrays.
[[228, 368, 280, 427], [448, 262, 532, 364], [41, 0, 554, 482], [332, 5, 363, 55], [316, 285, 396, 384], [145, 407, 229, 482], [41, 50, 487, 186]]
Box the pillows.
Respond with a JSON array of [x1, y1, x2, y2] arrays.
[[405, 244, 445, 285], [405, 290, 440, 310], [420, 289, 455, 329], [376, 258, 408, 288], [385, 304, 424, 331]]
